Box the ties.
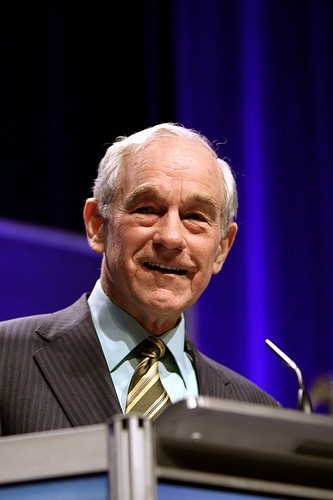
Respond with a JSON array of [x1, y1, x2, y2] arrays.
[[123, 336, 172, 421]]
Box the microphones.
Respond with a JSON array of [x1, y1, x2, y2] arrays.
[[263, 338, 313, 412]]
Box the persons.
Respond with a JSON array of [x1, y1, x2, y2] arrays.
[[1, 122, 282, 438]]
